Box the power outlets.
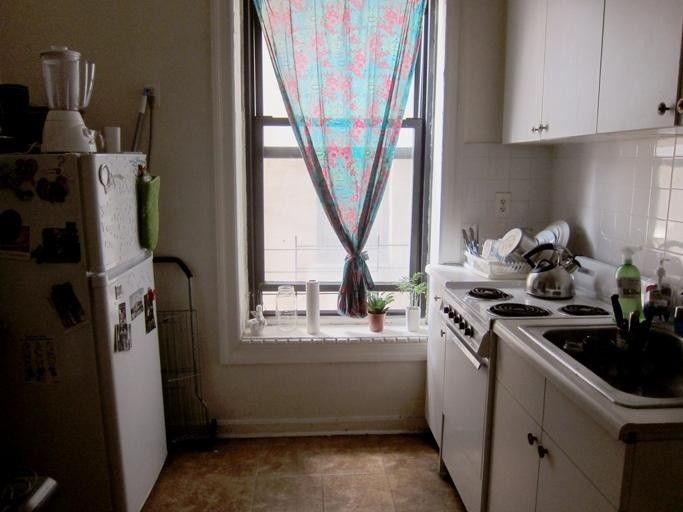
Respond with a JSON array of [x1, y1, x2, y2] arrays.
[[495, 192, 510, 217]]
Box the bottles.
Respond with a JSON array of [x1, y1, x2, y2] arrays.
[[612, 246, 641, 326], [304, 278, 322, 335], [274, 284, 298, 332]]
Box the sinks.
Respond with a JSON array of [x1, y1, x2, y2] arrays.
[[518, 317, 683, 408]]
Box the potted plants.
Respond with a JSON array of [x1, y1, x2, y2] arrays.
[[366, 290, 394, 332], [397, 272, 427, 331]]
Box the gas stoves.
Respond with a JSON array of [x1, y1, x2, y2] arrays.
[[436, 283, 616, 356]]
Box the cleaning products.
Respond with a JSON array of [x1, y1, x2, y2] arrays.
[[613, 245, 648, 323]]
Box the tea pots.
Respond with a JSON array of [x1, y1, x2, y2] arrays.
[[519, 244, 580, 301]]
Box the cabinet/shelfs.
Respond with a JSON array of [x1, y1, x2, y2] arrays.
[[486, 338, 627, 512], [425, 315, 488, 512], [596, 0, 683, 134], [502, 0, 605, 145]]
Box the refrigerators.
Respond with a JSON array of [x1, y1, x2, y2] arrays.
[[0, 151, 168, 512]]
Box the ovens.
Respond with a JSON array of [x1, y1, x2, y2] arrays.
[[440, 323, 494, 512]]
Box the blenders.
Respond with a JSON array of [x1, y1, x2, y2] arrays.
[[37, 43, 97, 156]]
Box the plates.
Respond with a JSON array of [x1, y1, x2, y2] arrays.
[[460, 214, 577, 268]]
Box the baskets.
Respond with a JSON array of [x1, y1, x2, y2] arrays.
[[460, 250, 530, 280]]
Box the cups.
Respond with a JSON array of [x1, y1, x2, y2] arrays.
[[98, 126, 122, 153]]
